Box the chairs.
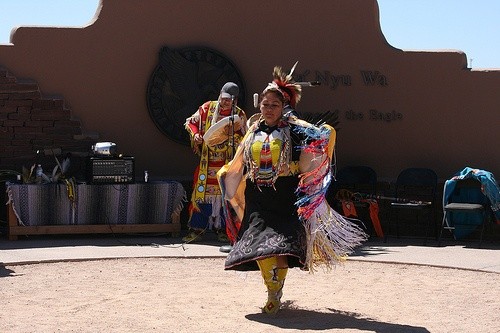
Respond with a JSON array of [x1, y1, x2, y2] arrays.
[[383, 166, 439, 246], [437, 176, 498, 249], [324, 165, 379, 230]]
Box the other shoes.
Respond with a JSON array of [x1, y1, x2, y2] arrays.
[[182, 227, 206, 243], [217, 229, 230, 243]]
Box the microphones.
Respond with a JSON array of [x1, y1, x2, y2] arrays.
[[231, 95, 236, 109]]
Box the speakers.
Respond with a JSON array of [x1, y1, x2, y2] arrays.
[[86, 155, 135, 184]]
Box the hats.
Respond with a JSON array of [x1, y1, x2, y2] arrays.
[[221, 82, 240, 98]]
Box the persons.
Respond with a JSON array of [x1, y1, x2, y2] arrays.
[[185, 81, 247, 243], [217, 81, 370, 316]]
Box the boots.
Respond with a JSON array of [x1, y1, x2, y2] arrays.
[[255, 256, 279, 315], [262, 267, 288, 313]]
